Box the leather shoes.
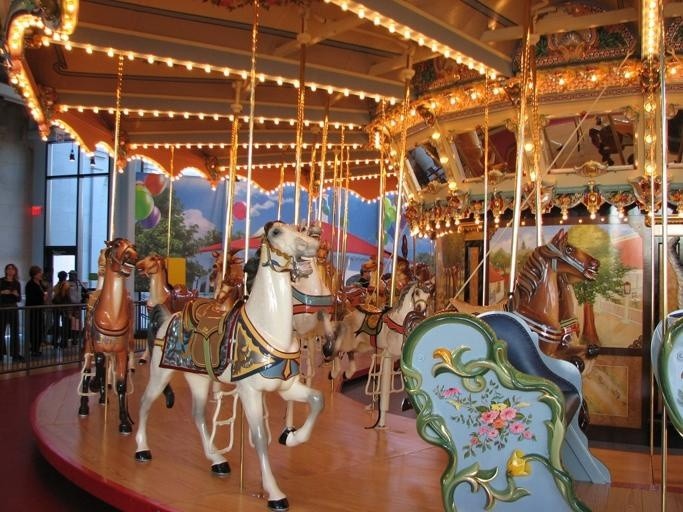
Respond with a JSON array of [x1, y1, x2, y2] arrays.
[[31, 351, 42, 357], [51, 338, 78, 350], [12, 355, 25, 362]]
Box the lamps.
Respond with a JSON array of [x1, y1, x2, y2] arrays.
[[70, 139, 97, 167]]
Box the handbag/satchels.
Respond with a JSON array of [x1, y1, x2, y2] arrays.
[[81, 286, 86, 302], [51, 283, 71, 305]]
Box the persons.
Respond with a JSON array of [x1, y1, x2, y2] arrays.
[[24, 265, 46, 358], [38, 272, 51, 349], [49, 270, 70, 349], [358, 269, 369, 288], [62, 270, 90, 345], [0, 264, 25, 362]]
